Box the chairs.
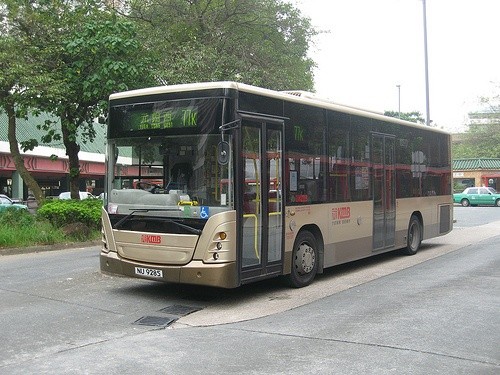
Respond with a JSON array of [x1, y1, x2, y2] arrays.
[[243, 182, 309, 214], [163, 161, 192, 194]]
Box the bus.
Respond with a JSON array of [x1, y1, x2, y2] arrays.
[[99, 81, 454, 288]]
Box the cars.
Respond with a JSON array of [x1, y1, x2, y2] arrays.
[[96, 193, 105, 200], [0, 194, 29, 213], [453, 186, 500, 207], [57, 192, 98, 201]]
